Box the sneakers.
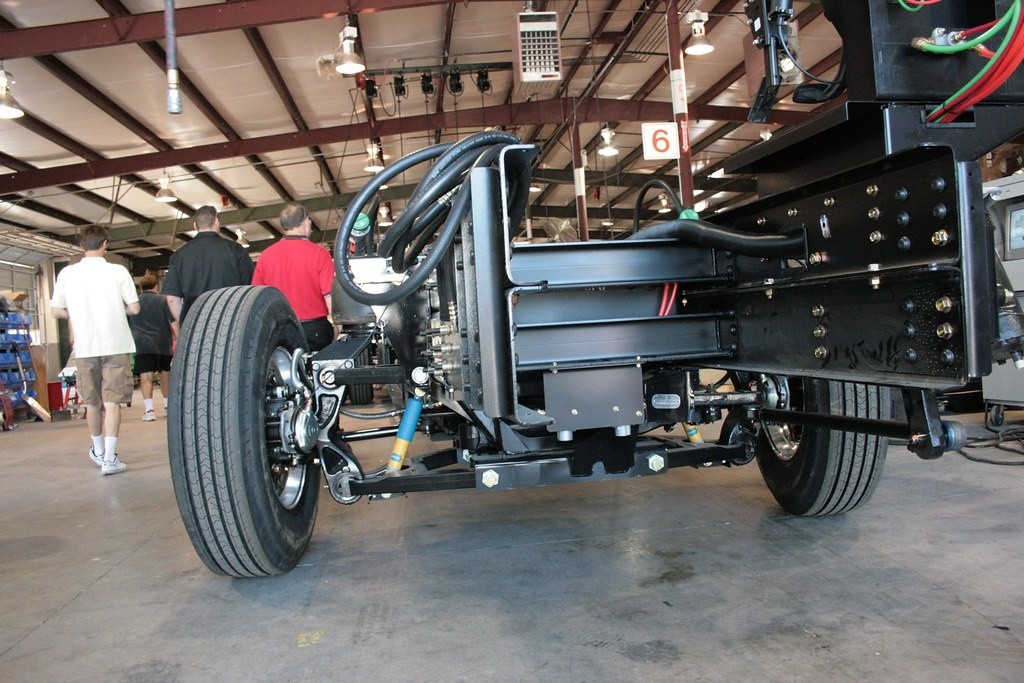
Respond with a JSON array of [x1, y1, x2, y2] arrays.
[[102, 453, 127, 475], [143, 410, 156, 420], [89, 447, 106, 467], [164, 408, 168, 417]]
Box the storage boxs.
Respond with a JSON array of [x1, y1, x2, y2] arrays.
[[0, 289, 30, 312]]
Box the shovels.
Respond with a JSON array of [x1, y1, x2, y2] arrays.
[[12, 343, 51, 425]]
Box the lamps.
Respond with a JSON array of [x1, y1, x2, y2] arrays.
[[364, 143, 385, 173], [602, 218, 614, 226], [155, 168, 177, 202], [598, 128, 618, 157], [530, 183, 541, 192], [235, 229, 250, 248], [659, 194, 671, 213], [335, 15, 365, 74], [685, 9, 714, 55], [378, 207, 392, 227], [365, 68, 490, 96], [0, 59, 24, 119]]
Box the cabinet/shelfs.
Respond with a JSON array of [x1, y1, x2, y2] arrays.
[[0, 308, 39, 429]]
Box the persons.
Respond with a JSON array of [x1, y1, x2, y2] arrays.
[[158, 204, 256, 321], [251, 202, 337, 355], [50, 224, 141, 475], [125, 273, 177, 420]]
